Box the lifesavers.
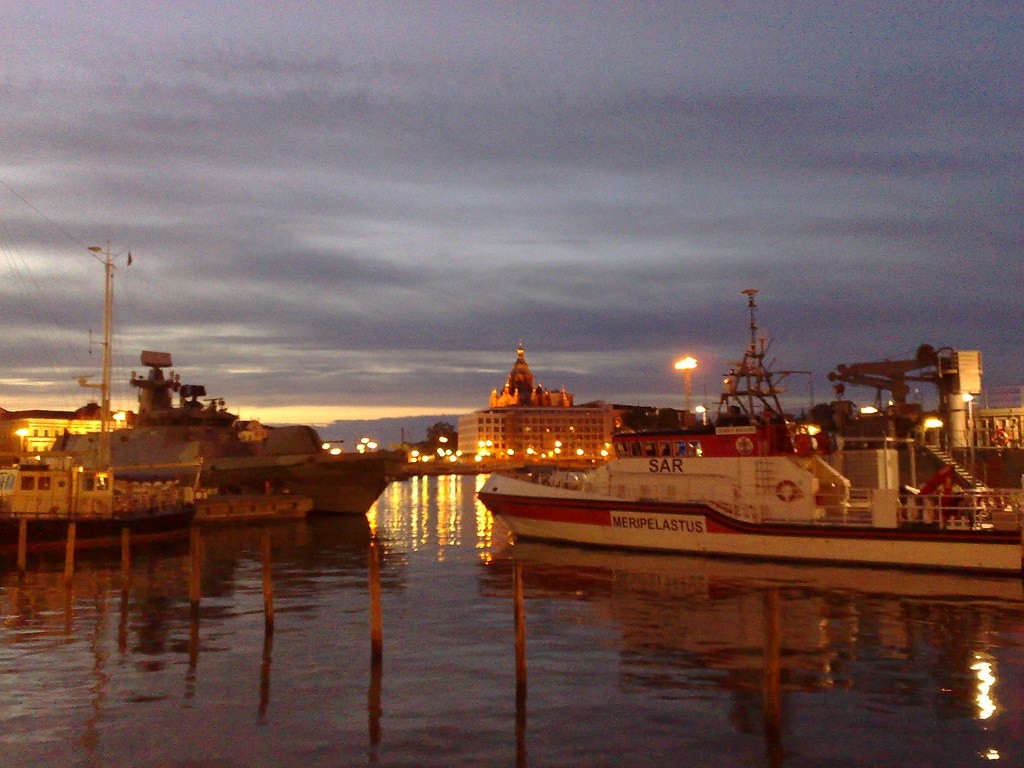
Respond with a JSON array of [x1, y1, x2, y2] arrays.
[[775, 480, 798, 503]]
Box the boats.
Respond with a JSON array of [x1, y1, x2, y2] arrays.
[[37, 349, 408, 516], [2, 238, 215, 518], [477, 287, 1024, 606]]
